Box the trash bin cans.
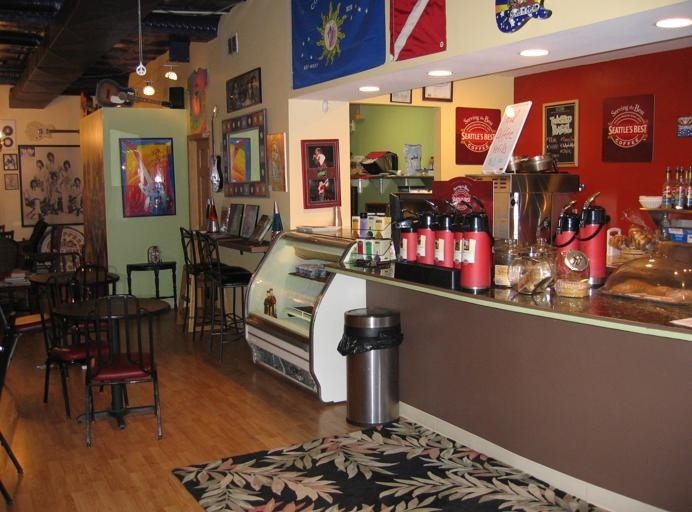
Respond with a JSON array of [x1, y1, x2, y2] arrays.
[[345, 307, 400, 428]]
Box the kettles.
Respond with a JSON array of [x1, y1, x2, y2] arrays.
[[454, 211, 464, 268], [579, 191, 611, 290], [553, 199, 579, 277], [416, 212, 435, 267], [460, 195, 492, 296], [434, 210, 454, 269], [398, 214, 417, 262]]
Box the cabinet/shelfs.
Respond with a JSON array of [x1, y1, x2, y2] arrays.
[[244, 228, 366, 404]]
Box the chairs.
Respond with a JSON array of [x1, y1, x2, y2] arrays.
[[29, 224, 64, 273], [179, 226, 229, 343], [0, 230, 14, 240], [0, 239, 18, 273], [16, 220, 48, 270], [68, 266, 116, 344], [0, 224, 5, 232], [49, 252, 83, 304], [85, 294, 163, 448], [34, 275, 128, 422], [194, 230, 252, 361], [0, 302, 68, 403]]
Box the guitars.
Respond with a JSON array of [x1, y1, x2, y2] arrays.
[[79, 89, 101, 116], [210, 115, 223, 192], [96, 78, 177, 108]]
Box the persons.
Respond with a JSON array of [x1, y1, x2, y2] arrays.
[[47, 173, 62, 209], [35, 159, 46, 180], [317, 178, 330, 201], [70, 178, 82, 211], [23, 179, 48, 212], [61, 160, 74, 177], [312, 147, 328, 171], [46, 152, 59, 171]]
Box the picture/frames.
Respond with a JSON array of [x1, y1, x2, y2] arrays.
[[542, 100, 579, 168], [229, 138, 251, 182], [390, 89, 412, 103], [3, 154, 18, 170], [227, 203, 244, 237], [17, 144, 85, 228], [118, 138, 177, 218], [300, 139, 341, 209], [4, 174, 19, 191], [265, 131, 288, 193], [226, 66, 262, 114], [423, 82, 453, 102], [240, 205, 259, 239]]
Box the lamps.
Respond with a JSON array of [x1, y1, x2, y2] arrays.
[[143, 81, 155, 96], [163, 64, 178, 81], [136, 0, 146, 76]]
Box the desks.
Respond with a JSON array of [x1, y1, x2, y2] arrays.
[[0, 284, 38, 315], [54, 295, 170, 430], [32, 270, 120, 366], [127, 262, 177, 311]]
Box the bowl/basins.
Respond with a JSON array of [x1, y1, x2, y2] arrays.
[[638, 196, 662, 209]]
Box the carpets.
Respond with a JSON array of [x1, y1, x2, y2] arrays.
[[171, 416, 610, 511]]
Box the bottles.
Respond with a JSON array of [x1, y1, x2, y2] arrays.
[[671, 167, 679, 205], [685, 166, 692, 209], [661, 213, 670, 241], [674, 167, 686, 209], [661, 166, 673, 209], [684, 169, 689, 195]]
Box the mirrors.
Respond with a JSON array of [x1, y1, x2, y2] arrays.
[[221, 109, 269, 200]]
